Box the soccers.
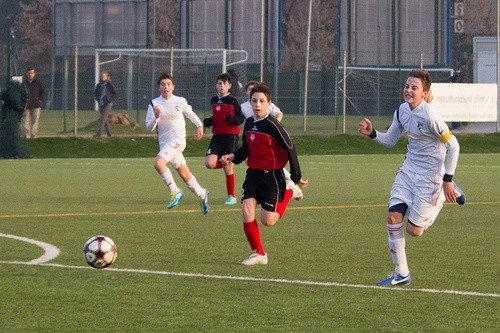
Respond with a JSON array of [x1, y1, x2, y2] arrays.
[[83, 235, 119, 269]]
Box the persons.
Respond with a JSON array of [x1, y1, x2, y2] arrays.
[[221, 83, 309, 266], [94, 69, 116, 138], [358, 70, 465, 286], [20, 66, 44, 139], [203, 69, 284, 206], [144, 73, 209, 215]]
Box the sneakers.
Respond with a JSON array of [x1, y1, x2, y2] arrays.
[[201, 188, 208, 214], [241, 252, 268, 265], [377, 274, 411, 286], [168, 191, 184, 209], [225, 195, 236, 204], [453, 180, 466, 205], [285, 180, 303, 202]]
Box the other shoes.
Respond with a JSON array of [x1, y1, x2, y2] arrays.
[[23, 134, 112, 138]]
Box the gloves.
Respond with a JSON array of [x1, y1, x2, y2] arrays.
[[203, 118, 211, 128], [225, 114, 237, 123]]
